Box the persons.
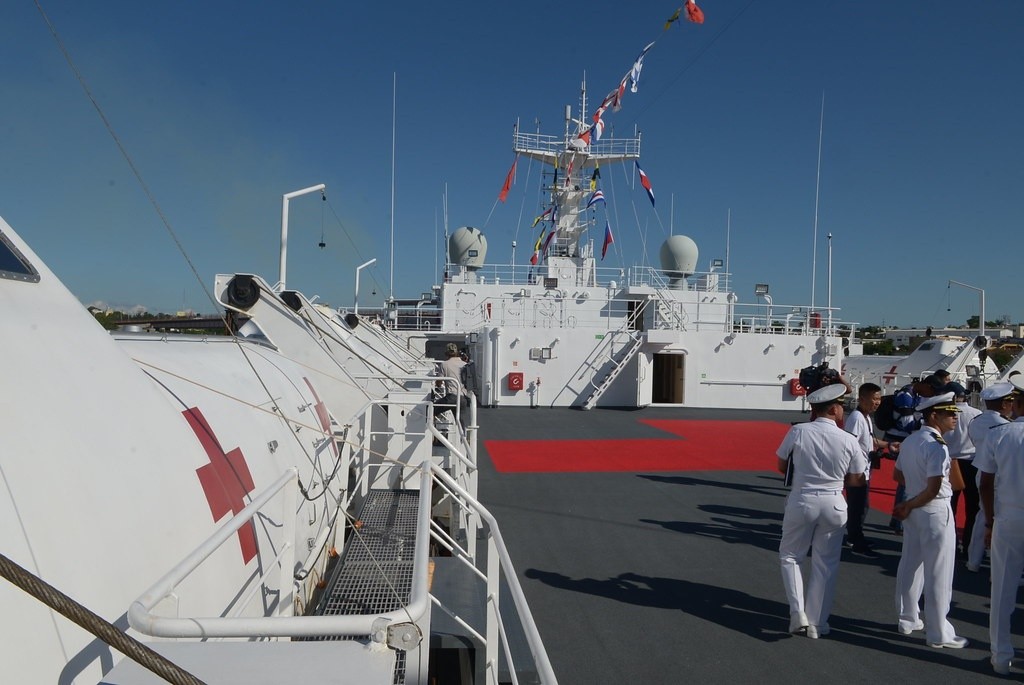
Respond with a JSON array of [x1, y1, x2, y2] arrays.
[[434, 344, 474, 432], [773, 383, 867, 642], [883, 368, 986, 556], [847, 381, 900, 558], [893, 391, 969, 649], [967, 371, 1024, 677], [800, 360, 851, 422]]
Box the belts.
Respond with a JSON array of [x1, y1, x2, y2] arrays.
[[801, 491, 842, 495]]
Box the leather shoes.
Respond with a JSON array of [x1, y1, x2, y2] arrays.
[[898, 619, 925, 634], [966, 561, 978, 572], [789, 612, 810, 635], [926, 636, 970, 649], [807, 622, 830, 639], [990, 655, 1012, 674]]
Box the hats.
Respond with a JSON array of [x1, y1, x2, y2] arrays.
[[981, 382, 1014, 402], [445, 343, 457, 354], [926, 375, 943, 396], [915, 391, 963, 415], [1009, 373, 1024, 394], [944, 381, 966, 397], [807, 383, 847, 408]]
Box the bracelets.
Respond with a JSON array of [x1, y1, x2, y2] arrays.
[[887, 441, 891, 451]]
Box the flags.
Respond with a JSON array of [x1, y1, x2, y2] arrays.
[[586, 188, 607, 207], [572, 50, 649, 148], [528, 226, 546, 264], [551, 203, 558, 227], [600, 220, 614, 261], [532, 208, 551, 229], [542, 230, 555, 257], [590, 158, 602, 193], [498, 153, 520, 202], [553, 156, 558, 188], [567, 154, 575, 177], [635, 160, 655, 207]]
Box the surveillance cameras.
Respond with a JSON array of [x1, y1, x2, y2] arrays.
[[512, 241, 517, 246], [827, 234, 832, 238]]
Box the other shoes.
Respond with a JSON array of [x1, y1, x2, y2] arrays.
[[963, 547, 988, 558], [847, 535, 874, 547], [890, 522, 896, 530], [956, 548, 961, 555], [895, 529, 903, 536], [851, 545, 882, 559]]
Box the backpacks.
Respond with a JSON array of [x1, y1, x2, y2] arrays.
[[878, 384, 915, 430]]
